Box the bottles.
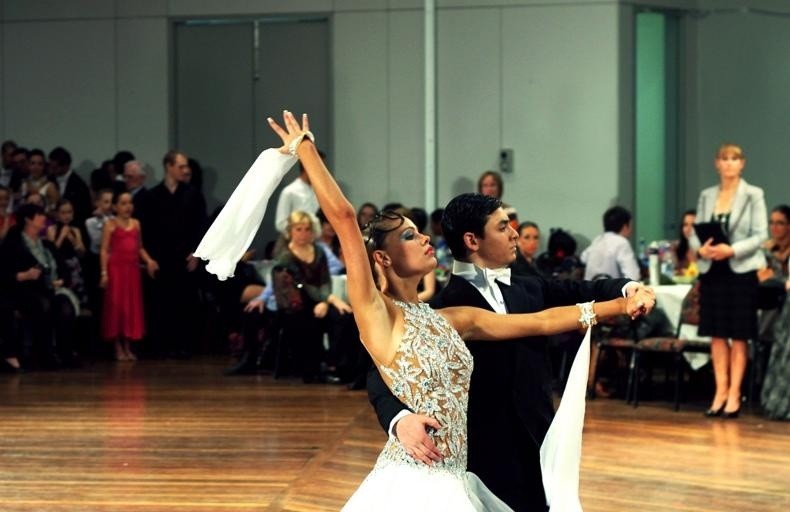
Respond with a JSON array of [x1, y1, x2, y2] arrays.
[[648, 247, 660, 286]]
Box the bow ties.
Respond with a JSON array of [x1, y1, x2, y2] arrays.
[[482, 265, 513, 289]]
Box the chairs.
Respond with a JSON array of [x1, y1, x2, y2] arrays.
[[589, 277, 773, 413]]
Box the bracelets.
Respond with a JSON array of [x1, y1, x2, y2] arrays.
[[289, 130, 315, 157]]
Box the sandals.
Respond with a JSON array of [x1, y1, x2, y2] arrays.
[[112, 348, 136, 362]]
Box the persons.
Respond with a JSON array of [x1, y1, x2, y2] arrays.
[[1, 134, 790, 424], [366, 191, 654, 511], [263, 108, 654, 511]]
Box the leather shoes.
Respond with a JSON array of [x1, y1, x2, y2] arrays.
[[706, 397, 743, 420], [323, 373, 341, 383], [223, 356, 256, 375], [348, 377, 369, 390]]
